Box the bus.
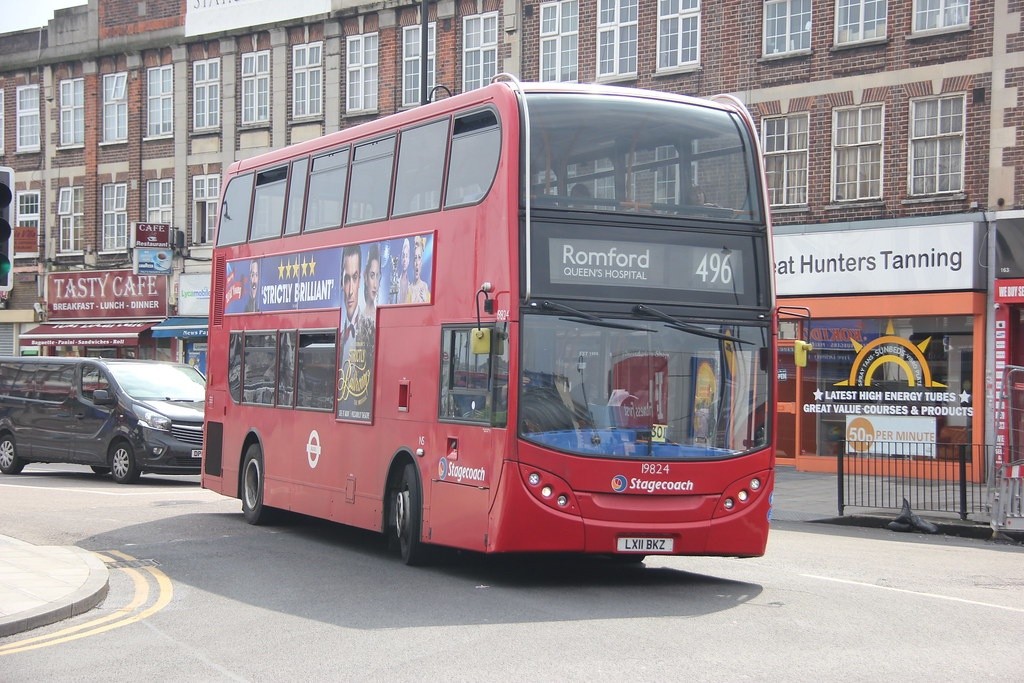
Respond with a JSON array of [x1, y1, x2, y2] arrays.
[[200, 71, 814, 568]]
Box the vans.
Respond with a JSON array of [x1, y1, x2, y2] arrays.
[[0, 356, 207, 485]]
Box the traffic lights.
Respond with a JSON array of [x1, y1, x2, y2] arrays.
[[0, 165, 15, 292]]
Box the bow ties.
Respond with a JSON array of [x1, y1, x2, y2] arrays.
[[344, 316, 359, 340]]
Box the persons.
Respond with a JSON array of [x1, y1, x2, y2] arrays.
[[567, 184, 592, 210], [685, 186, 720, 207], [245, 260, 260, 313], [339, 235, 430, 381]]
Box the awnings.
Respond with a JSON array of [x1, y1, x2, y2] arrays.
[[19, 316, 209, 345]]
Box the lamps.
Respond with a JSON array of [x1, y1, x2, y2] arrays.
[[33, 303, 46, 314]]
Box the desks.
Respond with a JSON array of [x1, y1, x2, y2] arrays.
[[820, 416, 846, 422]]
